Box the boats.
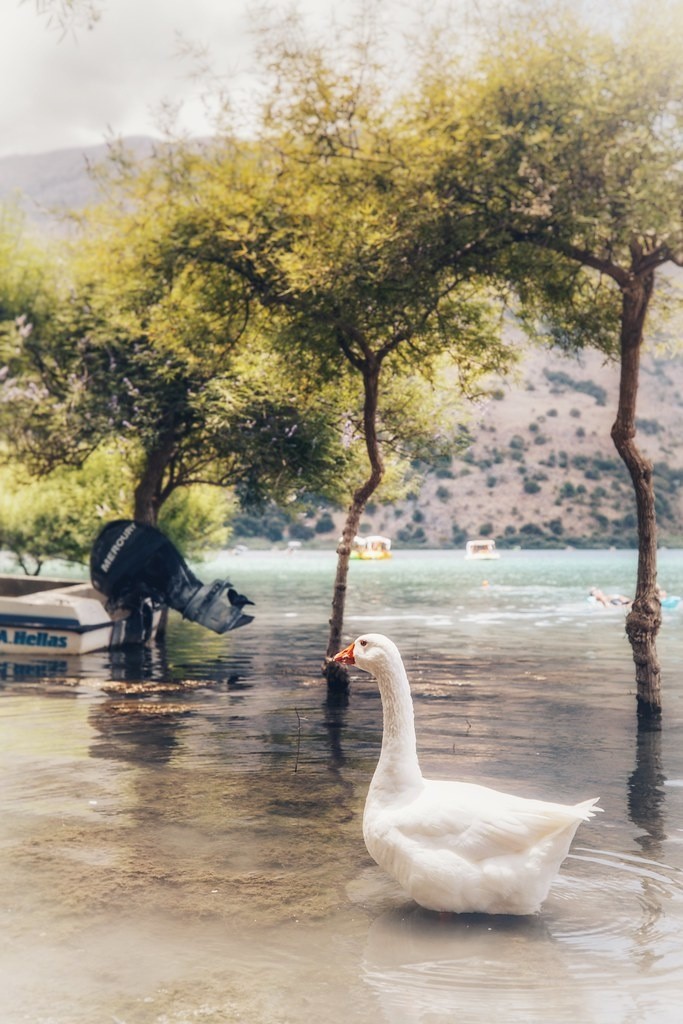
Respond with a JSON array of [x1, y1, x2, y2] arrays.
[[589, 594, 634, 609], [0, 518, 254, 657], [660, 596, 681, 610], [342, 537, 394, 559], [465, 541, 500, 560]]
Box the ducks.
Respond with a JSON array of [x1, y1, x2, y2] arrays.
[[333, 632, 605, 915]]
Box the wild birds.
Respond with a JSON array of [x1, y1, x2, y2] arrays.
[[592, 591, 633, 608]]
